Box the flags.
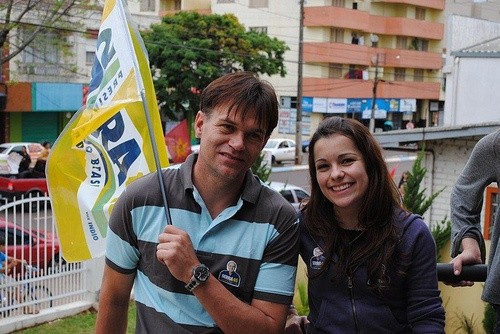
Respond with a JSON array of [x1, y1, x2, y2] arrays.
[[46, 0, 171, 262]]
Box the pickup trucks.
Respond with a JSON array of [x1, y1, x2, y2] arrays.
[[0, 158, 50, 213]]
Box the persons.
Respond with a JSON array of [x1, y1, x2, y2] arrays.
[[19, 146, 31, 173], [0, 235, 39, 314], [448, 128, 500, 334], [285, 115, 446, 334], [405, 121, 415, 129], [95, 71, 299, 334], [39, 142, 50, 161], [384, 117, 393, 131]]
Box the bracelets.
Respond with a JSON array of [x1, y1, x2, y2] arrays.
[[288, 305, 296, 309]]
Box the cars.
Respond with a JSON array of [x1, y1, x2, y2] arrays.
[[262, 138, 297, 165], [265, 181, 312, 215], [302, 139, 311, 153], [0, 216, 66, 279], [0, 141, 45, 176]]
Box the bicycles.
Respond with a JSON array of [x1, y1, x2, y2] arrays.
[[0, 260, 53, 318]]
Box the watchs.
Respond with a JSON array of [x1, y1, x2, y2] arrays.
[[185, 264, 210, 291]]
